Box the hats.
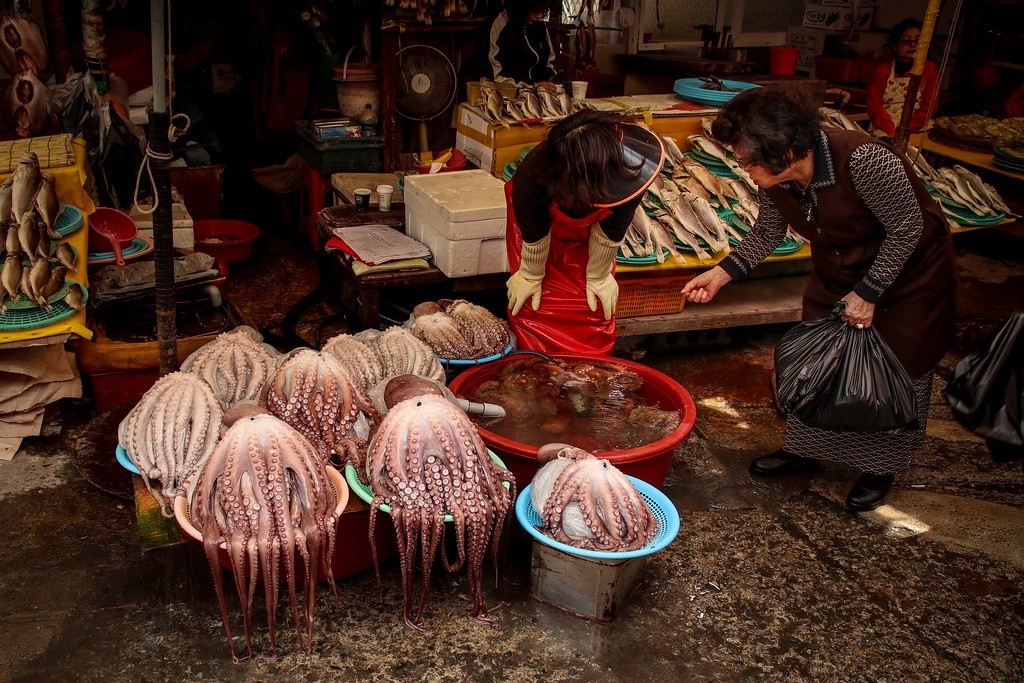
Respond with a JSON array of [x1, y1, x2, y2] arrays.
[[582, 120, 665, 208]]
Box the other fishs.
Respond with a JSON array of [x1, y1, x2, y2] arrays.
[[475, 76, 601, 132], [0, 150, 87, 316], [614, 104, 1022, 265]]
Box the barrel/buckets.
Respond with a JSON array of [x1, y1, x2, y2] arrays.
[[333, 45, 380, 116], [770, 48, 797, 75]]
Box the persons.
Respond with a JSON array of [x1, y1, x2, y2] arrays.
[[969, 46, 1014, 117], [867, 17, 939, 145], [505, 110, 665, 356], [682, 80, 959, 511], [467, 0, 557, 85], [250, 24, 309, 167]]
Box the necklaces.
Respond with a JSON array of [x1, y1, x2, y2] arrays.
[[793, 172, 814, 196]]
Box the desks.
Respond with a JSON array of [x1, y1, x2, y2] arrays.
[[314, 203, 406, 260], [330, 249, 509, 327]]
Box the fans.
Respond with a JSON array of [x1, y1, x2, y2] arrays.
[[395, 45, 458, 152]]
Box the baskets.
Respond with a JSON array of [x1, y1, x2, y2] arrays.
[[613, 272, 698, 317], [515, 470, 681, 558], [116, 417, 509, 550]]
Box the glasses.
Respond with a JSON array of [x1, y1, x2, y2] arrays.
[[732, 153, 756, 173], [897, 38, 918, 46]]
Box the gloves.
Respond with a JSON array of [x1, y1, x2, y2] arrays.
[[505, 229, 552, 317], [586, 221, 620, 321]]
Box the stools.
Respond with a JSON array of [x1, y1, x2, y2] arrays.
[[299, 162, 332, 252]]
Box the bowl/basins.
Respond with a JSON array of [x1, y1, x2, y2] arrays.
[[414, 161, 465, 174], [191, 219, 259, 262], [449, 351, 696, 494]]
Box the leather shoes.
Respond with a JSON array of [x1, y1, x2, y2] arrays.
[[846, 470, 894, 510], [750, 449, 821, 476]]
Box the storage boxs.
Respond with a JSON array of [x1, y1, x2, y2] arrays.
[[857, 59, 874, 82], [130, 202, 194, 248], [813, 55, 862, 83], [455, 105, 557, 175], [68, 294, 261, 414], [531, 541, 647, 624], [309, 117, 362, 138], [616, 268, 698, 319], [802, 4, 874, 30], [171, 163, 225, 219], [822, 0, 880, 7], [403, 169, 510, 278], [292, 120, 386, 176], [466, 81, 518, 107], [330, 172, 404, 206]]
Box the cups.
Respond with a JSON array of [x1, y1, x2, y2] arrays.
[[353, 188, 371, 214], [377, 185, 394, 211], [572, 81, 588, 99]]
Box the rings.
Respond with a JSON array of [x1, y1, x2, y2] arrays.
[[855, 324, 865, 329]]
